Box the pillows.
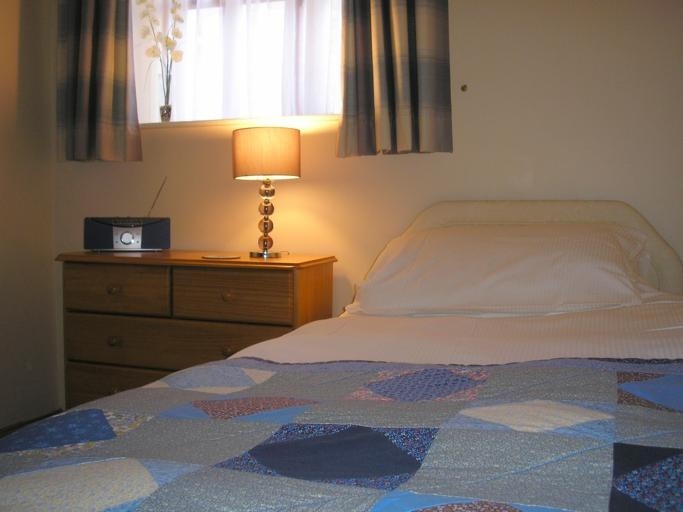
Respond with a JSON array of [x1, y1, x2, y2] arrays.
[[346, 221, 661, 315]]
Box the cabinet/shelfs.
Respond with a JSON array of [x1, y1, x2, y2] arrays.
[[52, 250, 338, 416]]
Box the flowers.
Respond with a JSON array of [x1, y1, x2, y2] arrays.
[[134, 0, 185, 105]]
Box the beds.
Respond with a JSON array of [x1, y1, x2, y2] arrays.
[[2, 198, 682, 511]]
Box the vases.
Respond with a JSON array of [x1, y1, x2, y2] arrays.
[[160, 106, 171, 122]]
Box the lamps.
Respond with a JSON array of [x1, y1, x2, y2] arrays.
[[233, 125, 301, 260]]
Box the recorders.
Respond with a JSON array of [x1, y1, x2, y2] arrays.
[[83, 216, 171, 251]]
